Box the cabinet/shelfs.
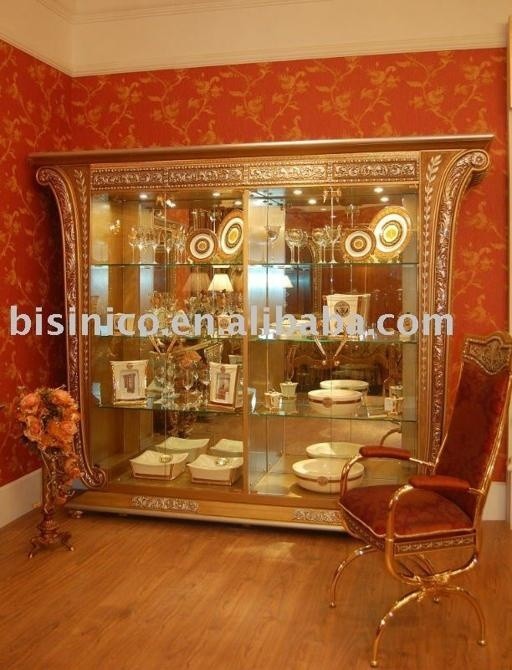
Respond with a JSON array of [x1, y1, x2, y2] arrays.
[[36, 149, 492, 547]]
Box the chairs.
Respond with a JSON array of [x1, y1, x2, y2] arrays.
[[329, 329, 512, 667]]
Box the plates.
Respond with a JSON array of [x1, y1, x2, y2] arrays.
[[342, 223, 377, 263], [183, 227, 219, 264], [366, 204, 414, 260], [212, 208, 244, 263]]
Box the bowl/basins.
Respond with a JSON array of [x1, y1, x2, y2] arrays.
[[318, 379, 370, 399], [306, 389, 364, 413], [292, 456, 365, 495], [305, 441, 365, 462]]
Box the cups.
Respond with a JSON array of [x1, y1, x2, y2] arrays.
[[280, 381, 299, 395], [264, 392, 283, 408]]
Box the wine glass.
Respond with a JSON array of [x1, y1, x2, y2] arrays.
[[147, 348, 214, 411], [264, 223, 341, 263], [123, 224, 191, 265]]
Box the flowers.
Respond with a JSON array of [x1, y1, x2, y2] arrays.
[[15, 386, 83, 508]]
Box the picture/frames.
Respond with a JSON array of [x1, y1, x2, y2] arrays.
[[109, 359, 150, 406], [205, 362, 239, 412]]
